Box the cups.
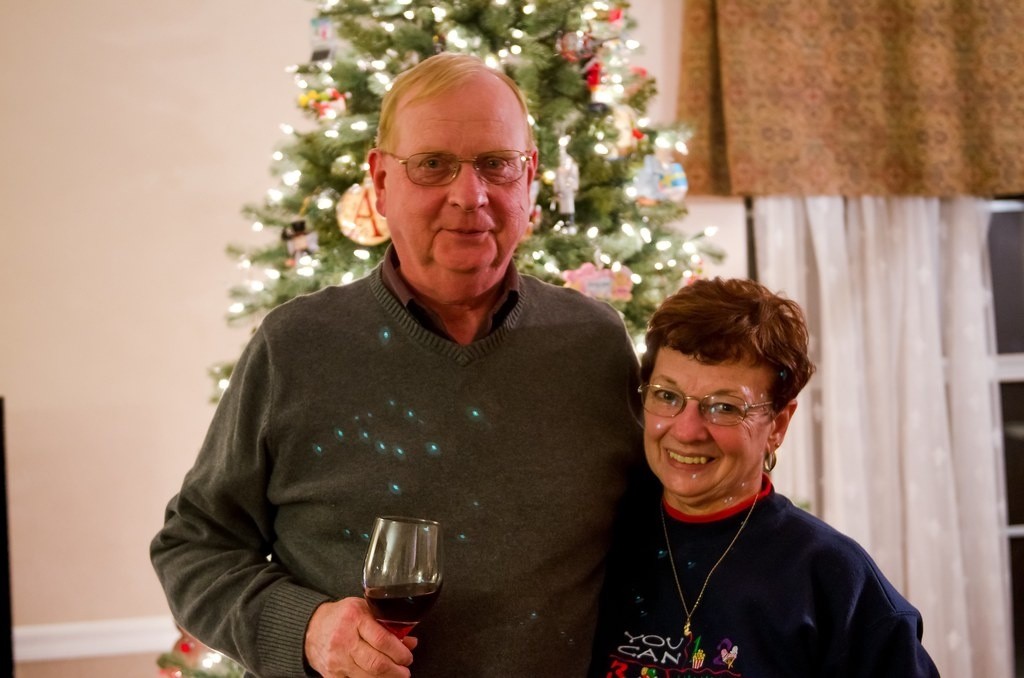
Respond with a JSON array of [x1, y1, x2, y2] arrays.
[[362, 514, 446, 640]]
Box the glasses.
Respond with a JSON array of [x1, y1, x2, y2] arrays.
[[377, 150, 532, 186], [636, 380, 776, 426]]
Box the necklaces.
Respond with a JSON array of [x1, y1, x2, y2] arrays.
[[661, 492, 758, 636]]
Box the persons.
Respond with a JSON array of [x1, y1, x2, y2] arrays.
[[597, 277, 939, 678], [151, 50, 640, 678]]
